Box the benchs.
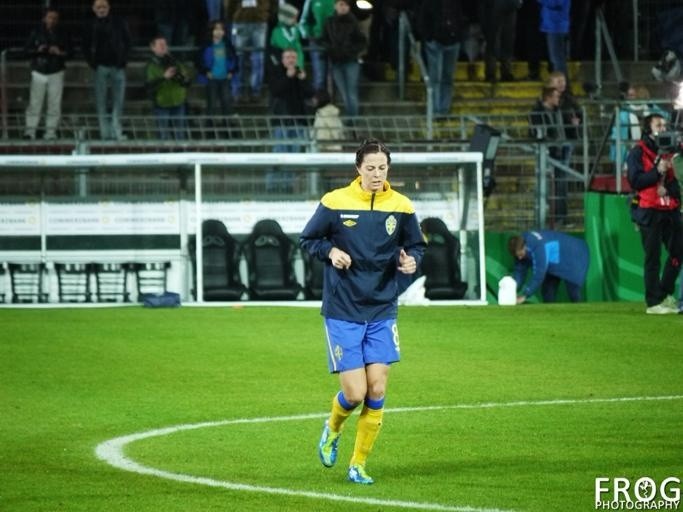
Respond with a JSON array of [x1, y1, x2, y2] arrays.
[[0, 36, 683, 231]]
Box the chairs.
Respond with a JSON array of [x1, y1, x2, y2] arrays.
[[302, 253, 324, 299], [130, 264, 171, 303], [189, 219, 242, 302], [8, 263, 48, 303], [93, 264, 130, 302], [421, 218, 470, 300], [55, 264, 93, 303], [241, 219, 301, 301]]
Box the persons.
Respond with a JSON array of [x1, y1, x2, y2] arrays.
[[23, 1, 682, 186], [298, 139, 426, 484], [626, 113, 683, 315], [508, 228, 591, 302]]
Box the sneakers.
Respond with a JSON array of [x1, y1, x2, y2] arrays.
[[347, 465, 374, 483], [318, 419, 341, 467], [646, 294, 680, 315]]
